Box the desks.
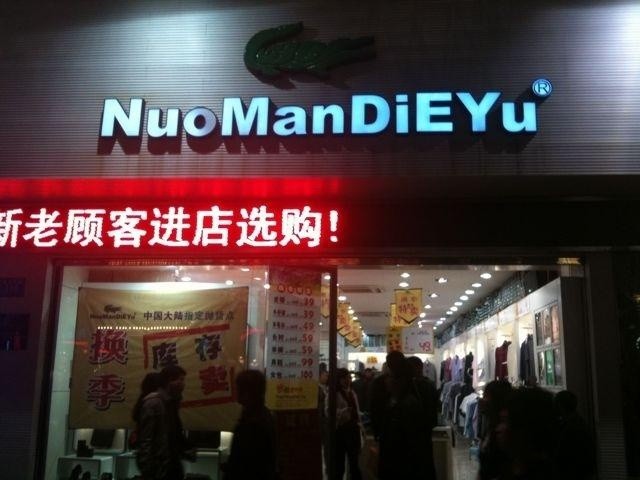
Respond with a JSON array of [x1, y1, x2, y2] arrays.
[[116, 451, 221, 480], [57, 454, 113, 480]]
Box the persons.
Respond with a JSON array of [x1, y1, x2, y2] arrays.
[[319, 350, 598, 480], [131, 372, 160, 423], [220, 370, 277, 479], [135, 364, 196, 479]]
